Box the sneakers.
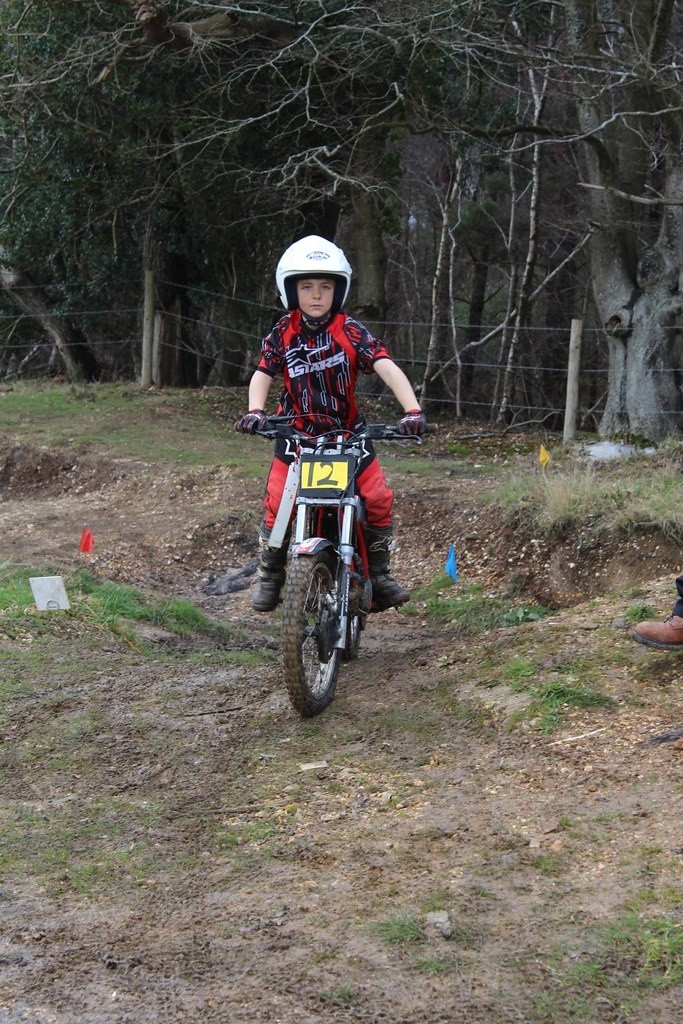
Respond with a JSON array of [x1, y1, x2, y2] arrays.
[[367, 568, 410, 609], [250, 566, 286, 612]]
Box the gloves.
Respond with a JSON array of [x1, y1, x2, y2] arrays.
[[234, 410, 269, 435], [397, 407, 426, 437]]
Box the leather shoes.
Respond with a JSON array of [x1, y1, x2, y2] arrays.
[[635, 613, 683, 648]]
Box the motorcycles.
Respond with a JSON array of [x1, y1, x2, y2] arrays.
[[235, 411, 440, 720]]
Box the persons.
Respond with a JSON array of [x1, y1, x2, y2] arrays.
[[630, 575, 683, 651], [234, 232, 433, 612]]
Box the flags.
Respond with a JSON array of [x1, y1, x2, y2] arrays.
[[444, 544, 459, 585], [79, 527, 95, 556], [538, 444, 550, 469]]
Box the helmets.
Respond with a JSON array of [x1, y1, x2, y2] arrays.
[[276, 235, 351, 314]]
